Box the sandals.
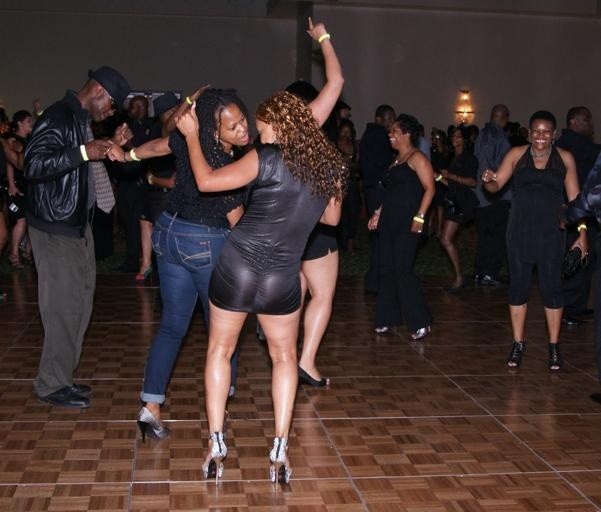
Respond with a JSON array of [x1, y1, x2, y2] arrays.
[[506, 341, 526, 367], [23, 252, 34, 265], [8, 254, 25, 269], [549, 342, 561, 371]]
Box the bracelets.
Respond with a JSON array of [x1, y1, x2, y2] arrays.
[[318, 33, 331, 42]]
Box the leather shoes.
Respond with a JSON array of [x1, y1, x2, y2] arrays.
[[446, 272, 502, 293], [560, 307, 594, 327], [40, 386, 91, 408], [298, 365, 326, 387], [69, 383, 93, 397]]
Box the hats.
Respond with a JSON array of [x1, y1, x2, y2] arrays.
[[88, 65, 130, 107], [153, 92, 182, 123]]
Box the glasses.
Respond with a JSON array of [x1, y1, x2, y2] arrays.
[[103, 88, 118, 110]]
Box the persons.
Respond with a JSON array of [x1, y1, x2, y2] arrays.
[[175, 17, 346, 490], [105, 89, 251, 444], [24, 66, 131, 409], [1, 81, 601, 412]]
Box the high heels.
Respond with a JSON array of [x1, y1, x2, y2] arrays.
[[136, 266, 153, 280], [269, 436, 292, 486], [408, 325, 432, 340], [201, 432, 228, 486], [137, 406, 170, 443], [373, 325, 398, 336]]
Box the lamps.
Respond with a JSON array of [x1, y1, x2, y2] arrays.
[[454, 89, 474, 125]]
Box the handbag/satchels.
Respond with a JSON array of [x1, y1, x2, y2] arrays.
[[560, 247, 588, 281]]
